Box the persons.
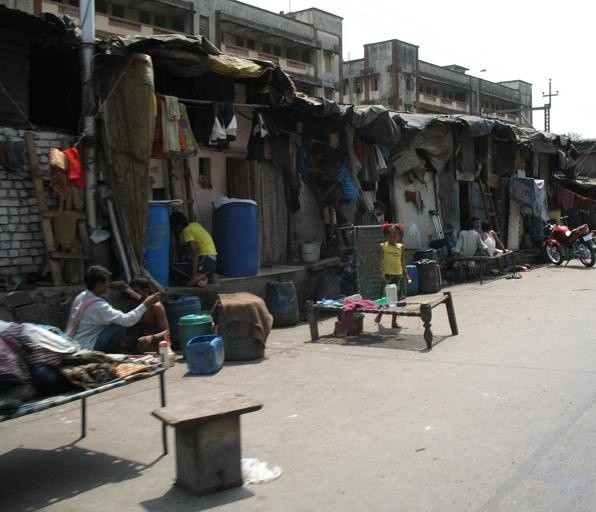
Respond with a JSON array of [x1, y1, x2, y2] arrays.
[[375, 224, 411, 329], [64, 266, 172, 355], [450, 217, 510, 260], [170, 211, 218, 288]]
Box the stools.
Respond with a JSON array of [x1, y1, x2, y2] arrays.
[[150, 393, 265, 497]]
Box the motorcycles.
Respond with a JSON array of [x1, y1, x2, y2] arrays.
[[541, 215, 596, 268]]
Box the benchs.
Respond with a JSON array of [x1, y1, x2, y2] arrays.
[[448, 248, 516, 286]]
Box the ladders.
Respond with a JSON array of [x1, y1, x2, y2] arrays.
[[21, 129, 97, 286], [477, 177, 503, 244]]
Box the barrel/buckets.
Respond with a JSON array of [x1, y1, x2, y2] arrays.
[[210, 202, 259, 277], [185, 334, 225, 375], [302, 242, 323, 263], [177, 314, 214, 359], [404, 265, 419, 295], [142, 204, 170, 288], [164, 296, 201, 350]]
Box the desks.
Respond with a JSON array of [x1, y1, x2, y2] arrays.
[[0, 352, 184, 455], [305, 291, 458, 350]]
